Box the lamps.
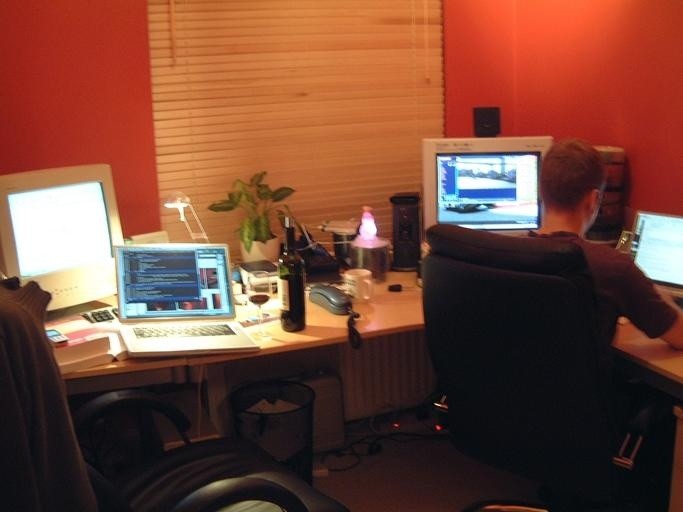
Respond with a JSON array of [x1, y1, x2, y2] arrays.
[[161, 191, 208, 242]]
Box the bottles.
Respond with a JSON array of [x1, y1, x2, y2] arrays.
[[388, 190, 419, 271], [275, 218, 307, 335]]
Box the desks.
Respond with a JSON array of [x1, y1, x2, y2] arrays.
[[0, 248, 683, 382]]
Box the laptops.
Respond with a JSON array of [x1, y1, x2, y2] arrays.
[[113, 243, 260, 359]]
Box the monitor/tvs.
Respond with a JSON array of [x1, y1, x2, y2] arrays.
[[0, 162, 125, 314], [626, 210, 683, 298], [422, 135, 553, 236]]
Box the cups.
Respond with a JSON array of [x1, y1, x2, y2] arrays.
[[330, 232, 355, 259], [348, 244, 388, 279], [344, 267, 376, 305]]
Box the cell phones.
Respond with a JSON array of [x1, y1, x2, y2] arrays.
[[45, 329, 70, 344]]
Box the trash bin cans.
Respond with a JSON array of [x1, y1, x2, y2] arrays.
[[232, 380, 315, 487]]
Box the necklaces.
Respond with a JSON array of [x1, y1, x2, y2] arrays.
[[526, 136, 683, 370]]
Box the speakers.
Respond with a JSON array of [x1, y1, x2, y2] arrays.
[[474, 107, 500, 137]]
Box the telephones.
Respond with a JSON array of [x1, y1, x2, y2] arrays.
[[309, 286, 351, 314]]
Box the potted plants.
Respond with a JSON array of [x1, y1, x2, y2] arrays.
[[208, 172, 295, 261]]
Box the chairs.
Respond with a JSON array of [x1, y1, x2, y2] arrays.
[[0, 292, 351, 512], [422, 223, 660, 511]]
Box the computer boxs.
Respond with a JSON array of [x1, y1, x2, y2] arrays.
[[70, 378, 210, 440]]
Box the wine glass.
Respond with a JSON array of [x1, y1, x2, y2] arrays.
[[244, 270, 274, 333]]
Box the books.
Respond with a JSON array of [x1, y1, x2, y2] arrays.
[[43, 314, 110, 363], [58, 320, 129, 374], [238, 259, 280, 295]]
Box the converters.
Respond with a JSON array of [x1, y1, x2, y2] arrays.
[[368, 443, 381, 455], [416, 405, 429, 420]]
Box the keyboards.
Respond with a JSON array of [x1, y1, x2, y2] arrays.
[[83, 306, 118, 323]]
